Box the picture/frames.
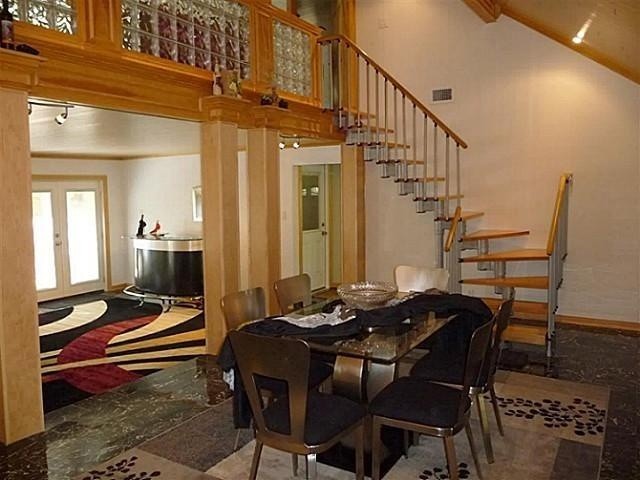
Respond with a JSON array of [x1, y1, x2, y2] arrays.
[[192, 187, 205, 223]]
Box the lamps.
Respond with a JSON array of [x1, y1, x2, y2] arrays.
[[55, 106, 68, 125]]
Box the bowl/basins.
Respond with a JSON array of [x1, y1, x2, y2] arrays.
[[336, 280, 400, 311]]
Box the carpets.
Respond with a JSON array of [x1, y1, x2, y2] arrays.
[[39, 291, 204, 416], [66, 368, 613, 480]]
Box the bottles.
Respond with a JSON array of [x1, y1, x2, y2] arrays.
[[0, 1, 15, 52], [212, 60, 245, 98]]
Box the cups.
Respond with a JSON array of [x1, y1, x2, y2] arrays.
[[260, 95, 289, 109]]
